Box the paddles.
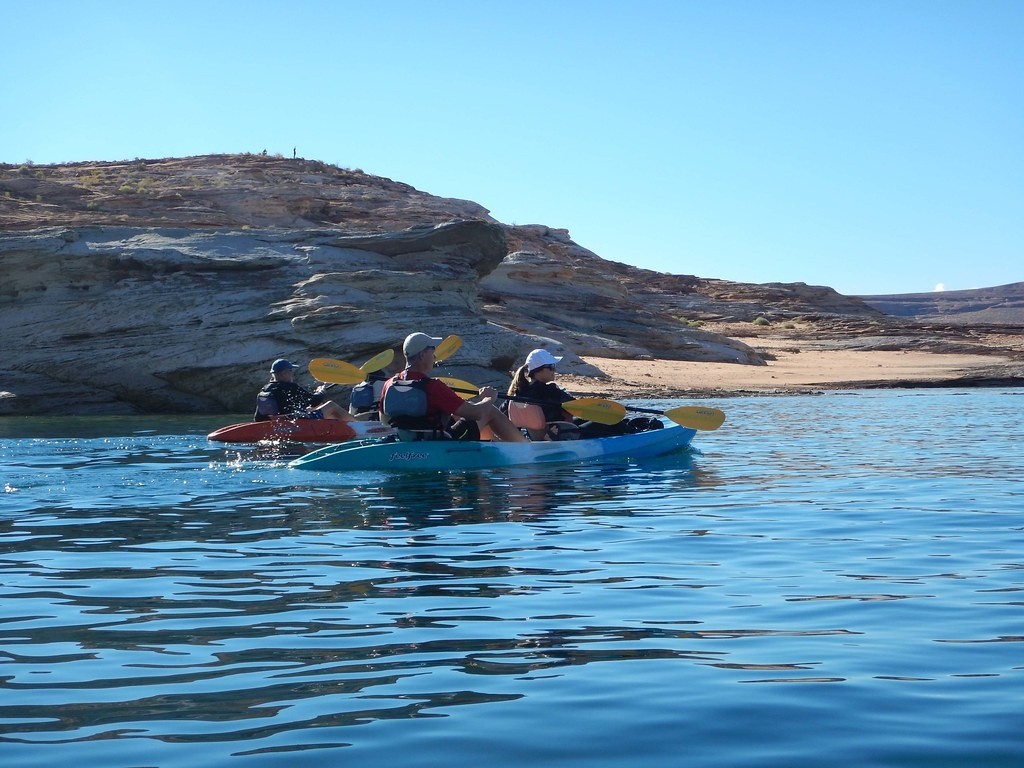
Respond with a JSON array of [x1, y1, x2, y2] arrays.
[[324, 348, 394, 389], [433, 334, 463, 365], [429, 376, 726, 431], [308, 358, 626, 425]]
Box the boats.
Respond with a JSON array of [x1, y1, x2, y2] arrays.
[[206, 413, 499, 445], [288, 409, 697, 475]]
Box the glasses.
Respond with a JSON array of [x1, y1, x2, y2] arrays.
[[543, 363, 557, 371]]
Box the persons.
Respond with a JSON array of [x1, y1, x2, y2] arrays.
[[379, 332, 530, 442], [255, 359, 371, 422], [348, 370, 390, 421], [499, 349, 664, 445]]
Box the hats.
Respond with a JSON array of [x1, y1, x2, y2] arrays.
[[403, 332, 443, 358], [270, 358, 299, 373], [525, 348, 563, 370]]
